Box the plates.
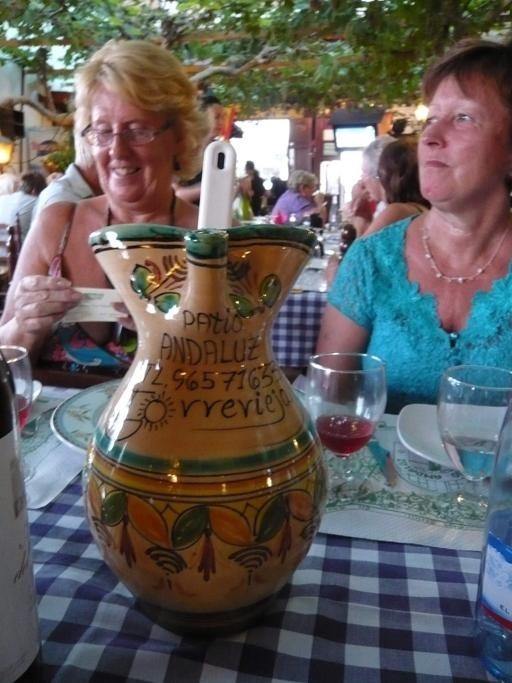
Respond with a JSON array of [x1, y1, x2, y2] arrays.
[[49, 377, 306, 463], [396, 403, 509, 472]]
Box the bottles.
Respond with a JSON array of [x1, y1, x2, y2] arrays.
[[474, 400, 512, 683], [82, 224, 327, 635], [0, 346, 44, 683]]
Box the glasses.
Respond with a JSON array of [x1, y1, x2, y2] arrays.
[[78, 117, 171, 144]]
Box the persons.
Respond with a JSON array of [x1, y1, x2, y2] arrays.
[[1, 96, 101, 275], [314, 38, 512, 414], [0, 38, 240, 372], [172, 97, 431, 285]]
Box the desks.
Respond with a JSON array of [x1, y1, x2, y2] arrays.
[[0, 194, 512, 682]]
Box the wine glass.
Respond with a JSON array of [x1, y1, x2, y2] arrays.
[[435, 365, 512, 521], [309, 351, 389, 507]]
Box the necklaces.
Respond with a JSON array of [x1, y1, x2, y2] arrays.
[[104, 187, 176, 288], [421, 223, 510, 283]]
